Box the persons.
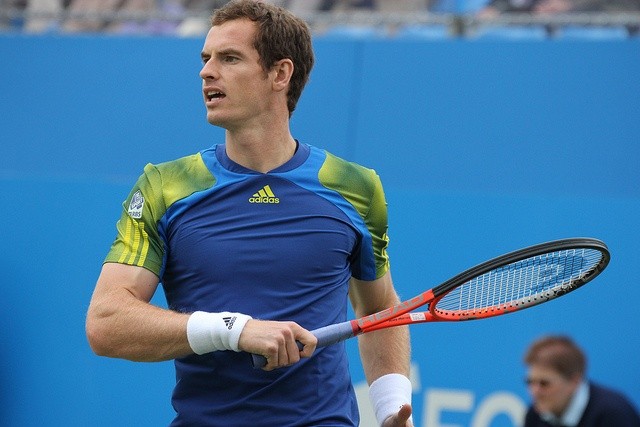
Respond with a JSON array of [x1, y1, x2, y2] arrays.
[[523, 333, 639, 427], [86, 0, 411, 427]]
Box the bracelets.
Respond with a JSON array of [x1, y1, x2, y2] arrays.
[[369, 373, 411, 427], [186, 309, 254, 356]]
